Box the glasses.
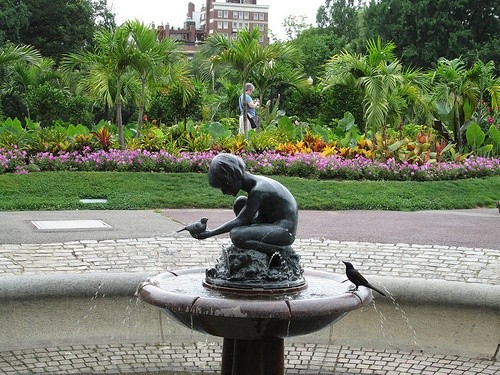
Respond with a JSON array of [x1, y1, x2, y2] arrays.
[[251, 90, 253, 92]]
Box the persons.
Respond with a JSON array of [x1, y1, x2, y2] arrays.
[[187, 152, 298, 260], [239, 82, 262, 134]]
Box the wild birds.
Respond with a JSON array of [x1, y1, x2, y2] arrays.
[[176, 217, 209, 236], [341, 260, 387, 298]]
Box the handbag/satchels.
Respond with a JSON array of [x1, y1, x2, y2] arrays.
[[250, 116, 258, 128]]
